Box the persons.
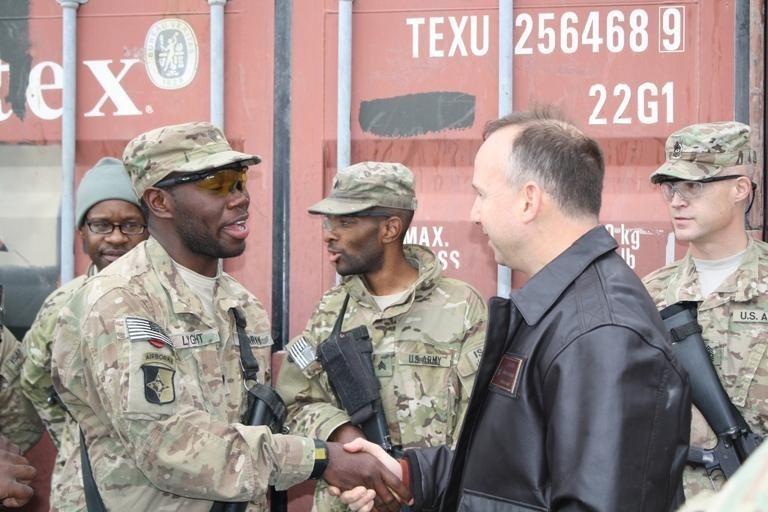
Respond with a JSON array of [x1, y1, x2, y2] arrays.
[[1, 432, 53, 510], [0, 235, 23, 373], [18, 157, 149, 510], [629, 120, 766, 512], [47, 123, 418, 511], [268, 161, 490, 511], [326, 103, 693, 511]]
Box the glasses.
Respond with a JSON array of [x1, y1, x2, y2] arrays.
[[156, 164, 249, 198], [84, 214, 147, 236], [656, 176, 743, 202]]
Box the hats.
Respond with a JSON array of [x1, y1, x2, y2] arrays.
[[121, 121, 263, 201], [649, 119, 756, 184], [72, 154, 149, 230], [306, 158, 420, 220]]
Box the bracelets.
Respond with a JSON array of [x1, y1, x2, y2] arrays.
[[309, 437, 328, 480]]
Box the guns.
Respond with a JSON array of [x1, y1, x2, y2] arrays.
[[652, 299, 764, 478], [316, 323, 396, 462], [212, 380, 288, 509]]
